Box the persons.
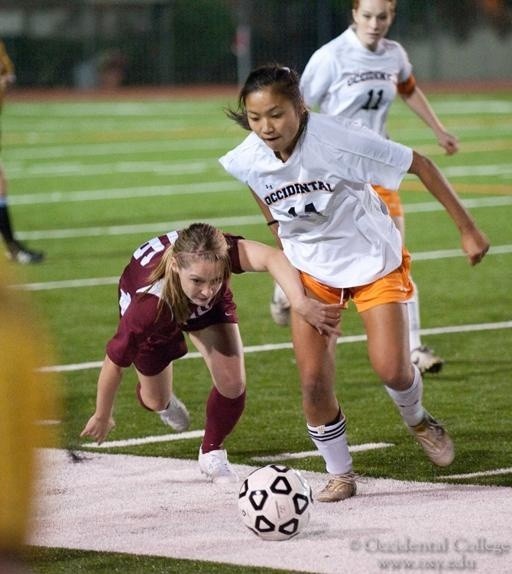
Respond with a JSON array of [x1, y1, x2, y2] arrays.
[[0, 41, 47, 268], [269, 0, 462, 375], [217, 59, 492, 504], [79, 220, 345, 487]]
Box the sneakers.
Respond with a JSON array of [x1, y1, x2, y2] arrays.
[[4, 242, 44, 265], [157, 393, 190, 433], [315, 470, 355, 503], [198, 444, 239, 486], [407, 407, 455, 466], [269, 281, 292, 327], [410, 346, 442, 375]]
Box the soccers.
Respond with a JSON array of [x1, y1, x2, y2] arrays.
[[236, 462, 314, 541]]
